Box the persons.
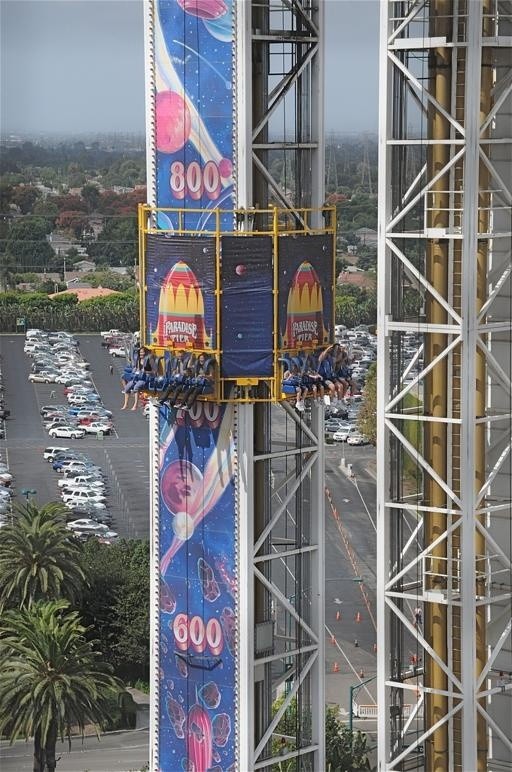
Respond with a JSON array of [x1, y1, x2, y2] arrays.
[[281, 343, 361, 413], [118, 346, 158, 411], [173, 353, 213, 410], [152, 350, 192, 409]]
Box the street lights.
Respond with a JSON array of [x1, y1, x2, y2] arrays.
[[345, 663, 418, 760], [283, 574, 363, 719], [22, 489, 38, 513]]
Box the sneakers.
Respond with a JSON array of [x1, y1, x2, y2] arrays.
[[173, 403, 183, 409], [164, 401, 173, 410], [294, 395, 355, 412], [153, 400, 164, 408], [180, 406, 192, 412]]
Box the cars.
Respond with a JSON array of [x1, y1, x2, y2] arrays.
[[43, 445, 121, 548], [24, 326, 117, 442], [100, 328, 151, 420], [1, 352, 16, 534], [323, 323, 425, 448]]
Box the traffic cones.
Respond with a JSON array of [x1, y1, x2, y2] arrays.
[[336, 611, 341, 622], [331, 635, 337, 645], [333, 662, 339, 674], [360, 667, 364, 678], [412, 654, 417, 663], [357, 612, 361, 624], [373, 642, 377, 653]]
